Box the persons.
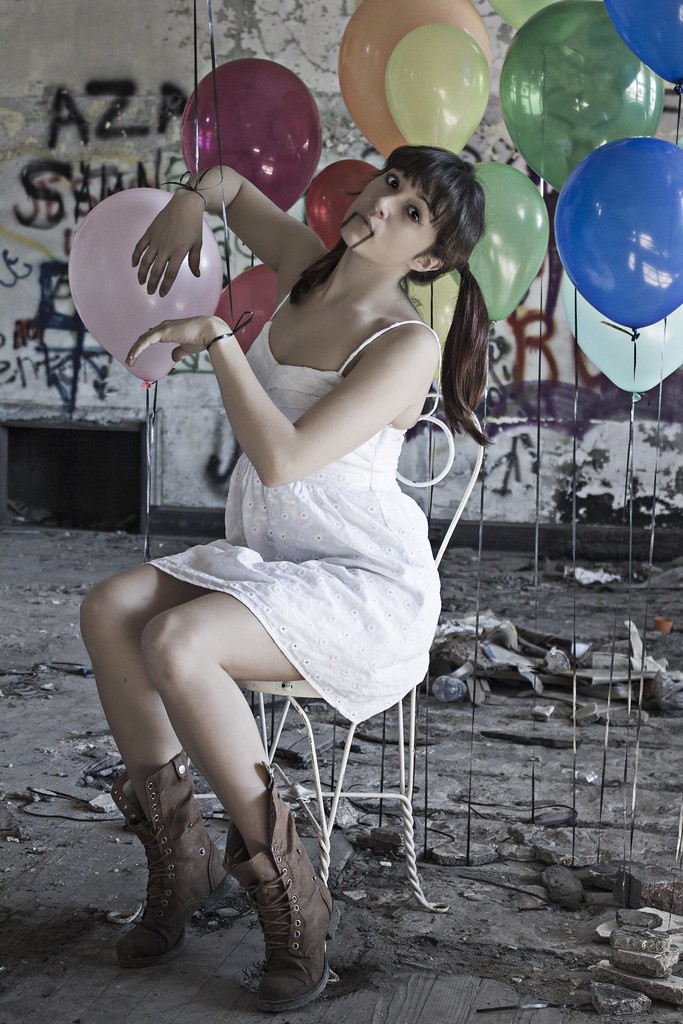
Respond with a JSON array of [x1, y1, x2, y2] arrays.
[[79, 145, 491, 1012]]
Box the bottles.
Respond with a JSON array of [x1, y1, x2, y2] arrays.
[[432, 676, 467, 703]]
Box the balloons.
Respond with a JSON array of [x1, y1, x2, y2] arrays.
[[67, 0, 683, 389]]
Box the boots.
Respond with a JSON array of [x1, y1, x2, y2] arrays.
[[222, 761, 340, 1012], [110, 748, 240, 967]]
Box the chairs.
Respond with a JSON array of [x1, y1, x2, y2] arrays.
[[193, 394, 485, 913]]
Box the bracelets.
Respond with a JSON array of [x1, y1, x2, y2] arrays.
[[164, 165, 223, 208], [203, 312, 255, 352]]
